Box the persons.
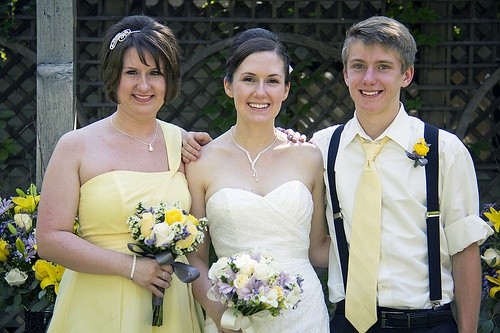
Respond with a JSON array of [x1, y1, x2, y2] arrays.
[[185, 28, 332, 333], [36, 15, 307, 333], [180, 17, 494, 333]]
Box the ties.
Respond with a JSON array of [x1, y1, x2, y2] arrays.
[[344, 134, 391, 333]]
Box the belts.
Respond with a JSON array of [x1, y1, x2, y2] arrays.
[[369, 307, 453, 330]]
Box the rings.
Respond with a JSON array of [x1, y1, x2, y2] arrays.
[[160, 269, 163, 279]]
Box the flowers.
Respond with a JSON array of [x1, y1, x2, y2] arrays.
[[479, 203, 500, 298], [0, 184, 79, 311], [405, 138, 431, 168], [207, 251, 305, 332], [126, 201, 208, 327]]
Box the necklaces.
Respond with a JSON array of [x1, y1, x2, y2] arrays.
[[110, 115, 159, 153], [230, 125, 279, 182]]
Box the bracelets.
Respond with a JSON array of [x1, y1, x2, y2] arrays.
[[130, 254, 137, 280]]
